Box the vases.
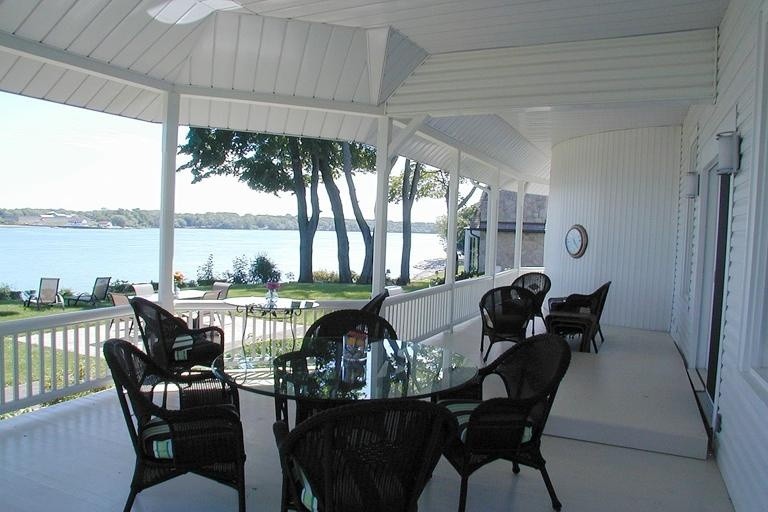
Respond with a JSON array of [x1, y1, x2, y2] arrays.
[[265, 289, 279, 301]]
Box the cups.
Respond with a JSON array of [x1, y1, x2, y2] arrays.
[[342, 335, 368, 362]]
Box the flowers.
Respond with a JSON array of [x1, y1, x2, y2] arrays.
[[266, 283, 281, 291]]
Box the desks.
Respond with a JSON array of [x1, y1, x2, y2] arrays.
[[223, 296, 319, 364], [211, 336, 480, 427]]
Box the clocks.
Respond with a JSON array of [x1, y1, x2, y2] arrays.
[[564, 225, 587, 258]]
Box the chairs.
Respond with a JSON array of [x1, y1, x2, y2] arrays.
[[432, 332, 572, 512], [480, 272, 611, 363], [131, 281, 233, 328], [128, 297, 225, 407], [272, 398, 458, 511], [104, 339, 246, 512], [24, 276, 111, 311], [307, 309, 397, 339], [360, 289, 390, 316]]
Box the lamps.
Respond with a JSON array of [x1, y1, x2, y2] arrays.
[[685, 170, 700, 200], [714, 130, 741, 176]]
